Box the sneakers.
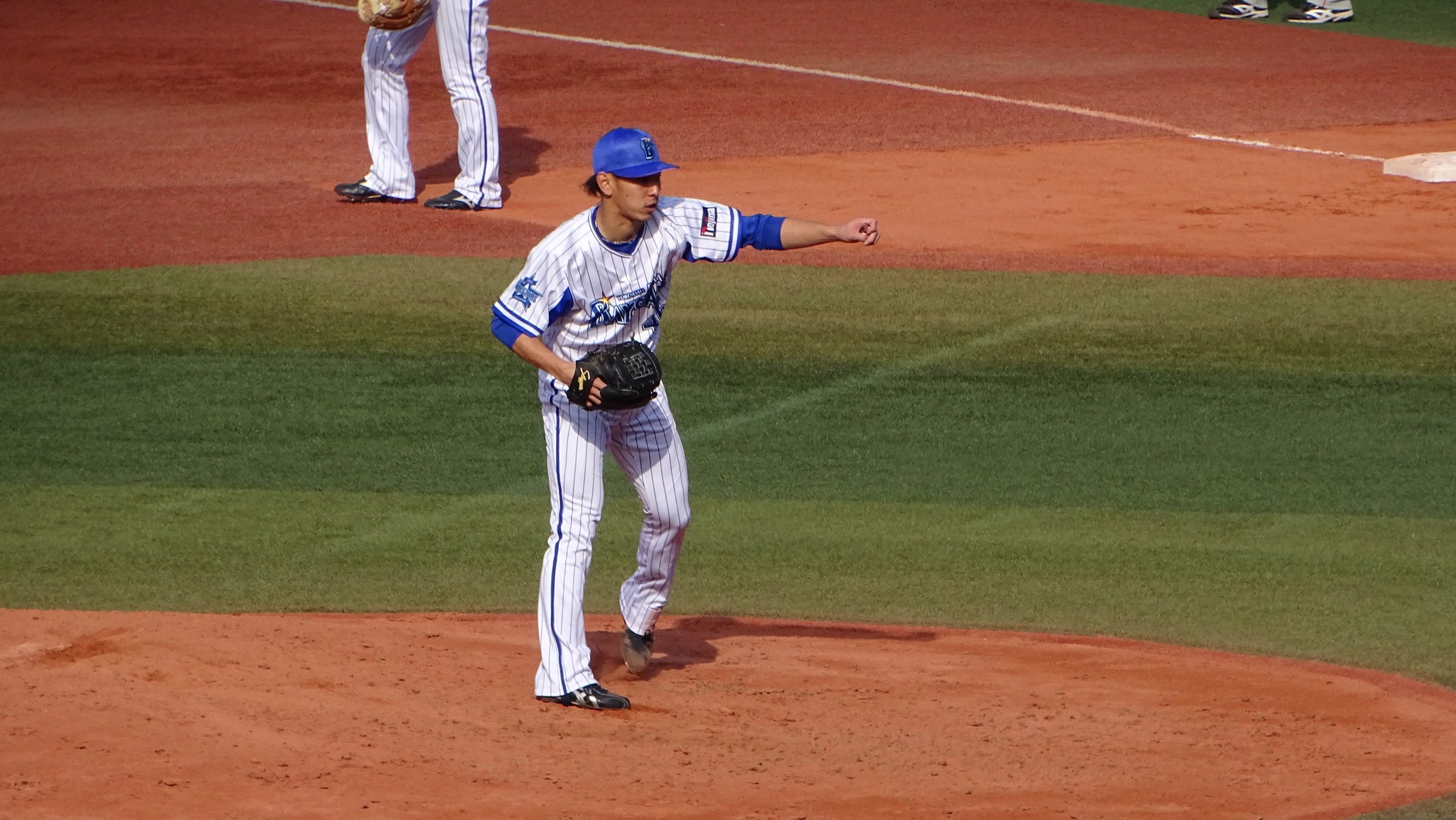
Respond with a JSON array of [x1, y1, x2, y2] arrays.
[[1207, 0, 1269, 20], [537, 683, 630, 710], [621, 616, 654, 673], [333, 178, 411, 202], [424, 190, 490, 211], [1285, 3, 1353, 21]]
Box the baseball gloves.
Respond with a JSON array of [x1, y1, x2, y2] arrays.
[[357, 0, 429, 30], [566, 340, 662, 413]]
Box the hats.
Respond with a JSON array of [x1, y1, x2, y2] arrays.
[[592, 126, 679, 178]]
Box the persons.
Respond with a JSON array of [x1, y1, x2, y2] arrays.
[[335, 0, 504, 208], [493, 128, 878, 710], [1208, 0, 1353, 23]]
